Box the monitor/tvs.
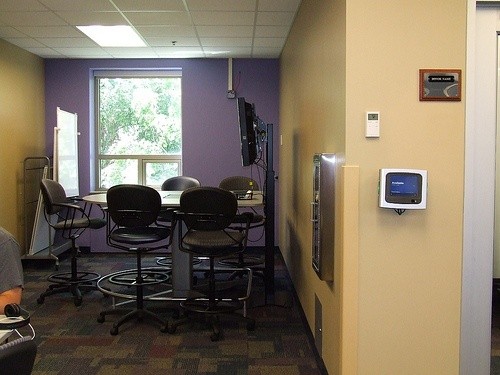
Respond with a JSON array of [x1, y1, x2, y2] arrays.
[[236, 97, 257, 167]]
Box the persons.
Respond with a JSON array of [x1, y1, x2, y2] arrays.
[[0, 227, 25, 314]]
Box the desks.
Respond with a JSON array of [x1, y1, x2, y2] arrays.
[[82, 191, 262, 317]]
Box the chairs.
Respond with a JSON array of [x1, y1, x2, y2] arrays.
[[36, 178, 110, 307], [99, 176, 263, 342]]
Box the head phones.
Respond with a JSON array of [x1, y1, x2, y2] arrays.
[[0, 303, 30, 330]]
